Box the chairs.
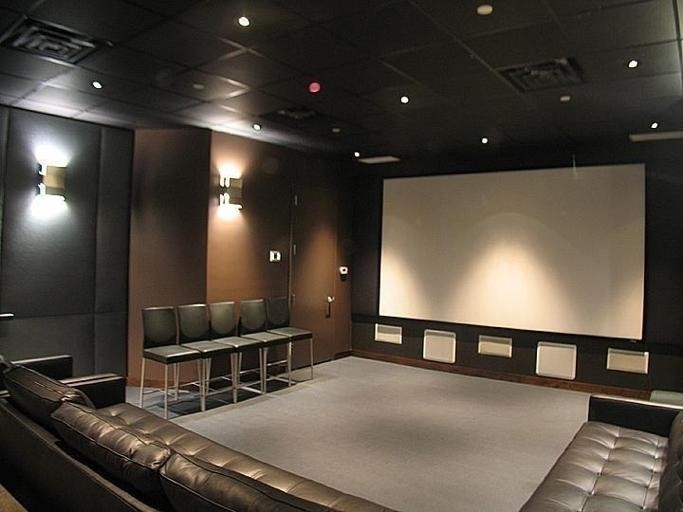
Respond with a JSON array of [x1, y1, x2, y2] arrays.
[[138, 296, 316, 420]]
